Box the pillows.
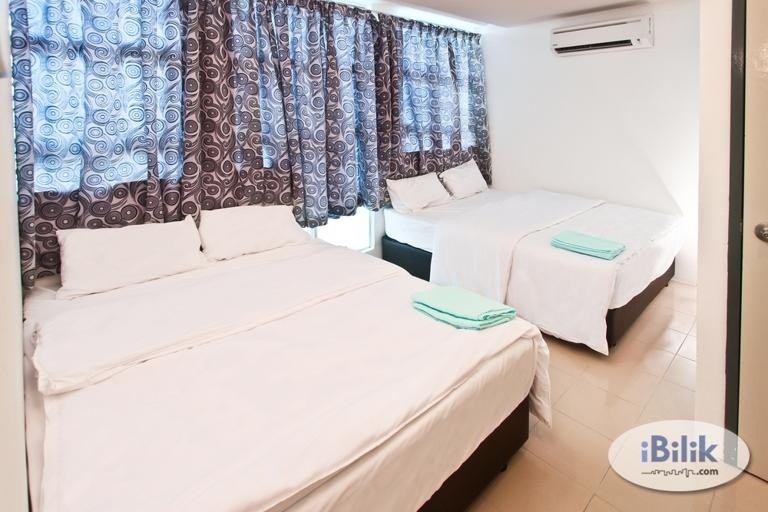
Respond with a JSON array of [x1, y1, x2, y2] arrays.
[[386, 159, 488, 214]]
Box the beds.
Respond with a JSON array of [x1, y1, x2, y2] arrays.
[[23, 178, 676, 512]]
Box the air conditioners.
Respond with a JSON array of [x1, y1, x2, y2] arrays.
[[550, 12, 654, 56]]
